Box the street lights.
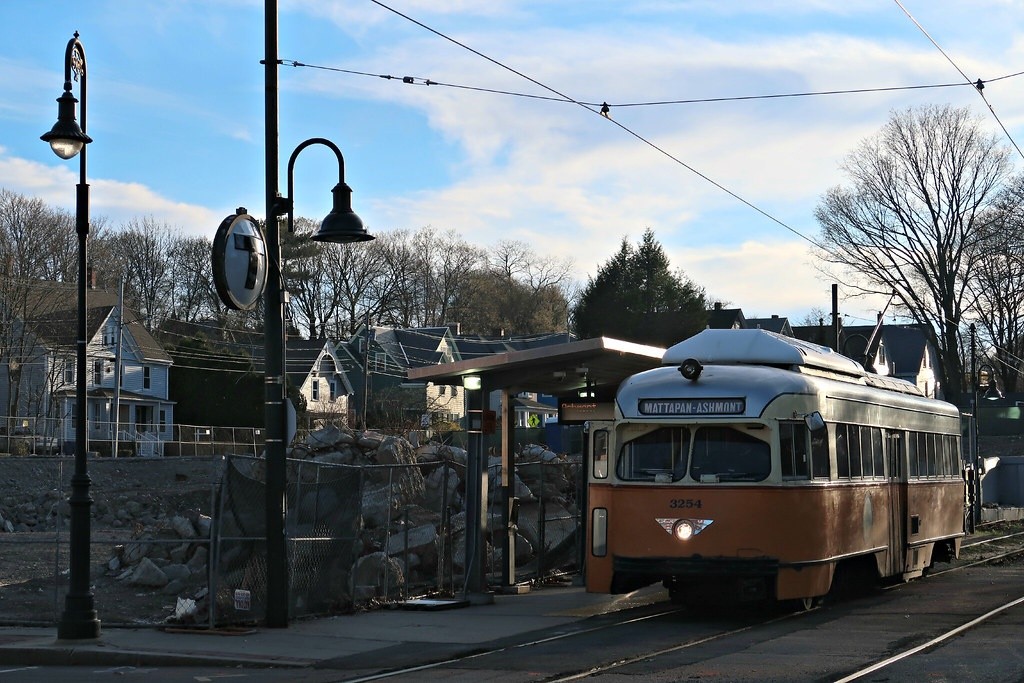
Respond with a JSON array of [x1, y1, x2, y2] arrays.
[[972, 364, 1006, 527], [260, 137, 377, 629], [40, 31, 103, 641]]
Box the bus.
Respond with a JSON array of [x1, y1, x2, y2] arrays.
[[585, 328, 968, 614]]
